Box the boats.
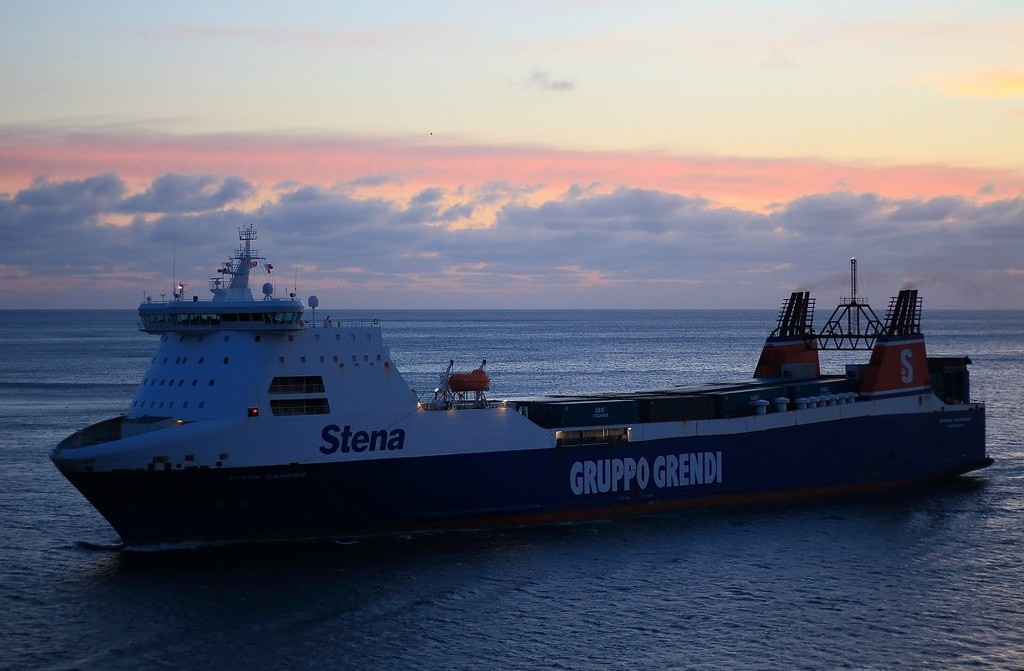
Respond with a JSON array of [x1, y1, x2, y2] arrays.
[[46, 224, 994, 563]]
